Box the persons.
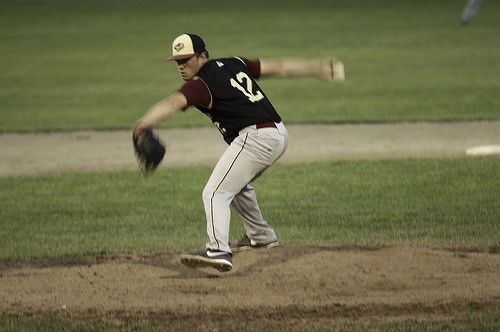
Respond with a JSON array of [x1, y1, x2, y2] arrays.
[[132, 33, 345, 272]]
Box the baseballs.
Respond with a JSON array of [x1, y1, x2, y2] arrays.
[[333, 61, 345, 83]]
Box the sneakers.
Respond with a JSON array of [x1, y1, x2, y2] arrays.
[[229, 233, 280, 252], [181, 246, 234, 272]]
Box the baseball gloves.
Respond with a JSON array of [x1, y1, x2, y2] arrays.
[[130, 128, 166, 181]]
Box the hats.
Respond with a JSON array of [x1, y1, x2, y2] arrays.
[[165, 32, 206, 62]]
